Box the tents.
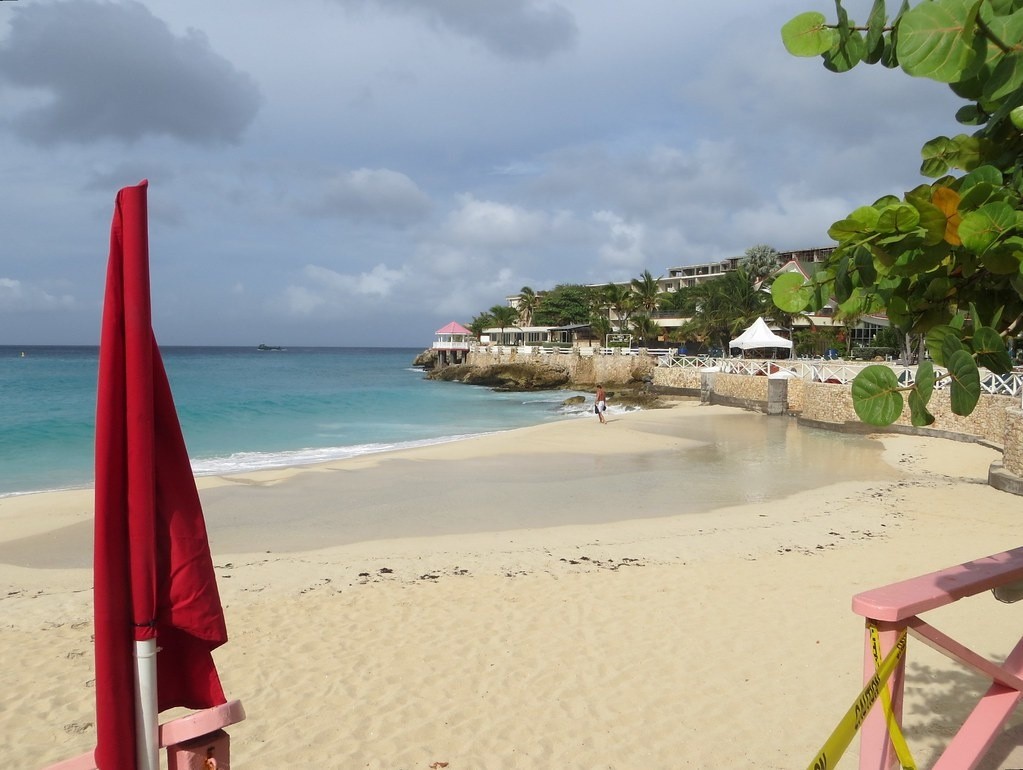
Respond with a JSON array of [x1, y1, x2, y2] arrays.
[[728, 317, 794, 360]]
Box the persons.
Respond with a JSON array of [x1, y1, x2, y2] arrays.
[[595, 385, 608, 425]]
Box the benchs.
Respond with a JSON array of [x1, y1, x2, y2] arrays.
[[756, 366, 780, 376]]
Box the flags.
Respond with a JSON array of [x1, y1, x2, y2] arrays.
[[92, 178, 227, 770]]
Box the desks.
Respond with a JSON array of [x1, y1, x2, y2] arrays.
[[769, 369, 792, 379], [700, 365, 730, 372]]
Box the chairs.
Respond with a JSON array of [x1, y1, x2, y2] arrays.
[[896, 369, 1023, 394]]
